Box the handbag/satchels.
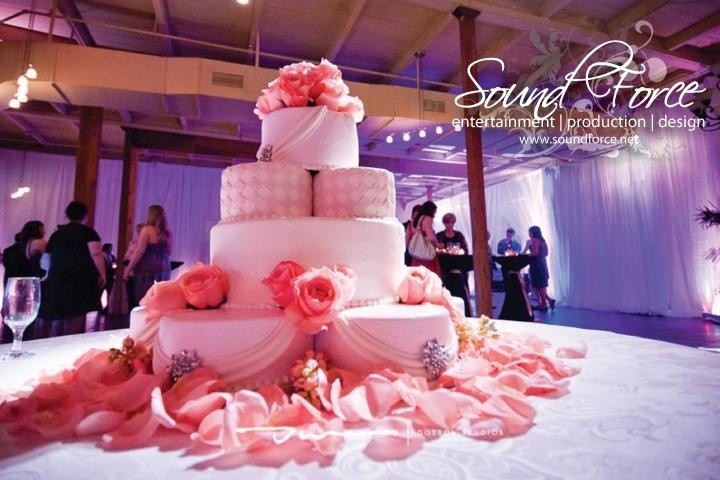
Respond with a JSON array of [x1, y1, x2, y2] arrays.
[[408, 231, 436, 260]]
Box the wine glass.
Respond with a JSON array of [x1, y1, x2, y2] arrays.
[[0, 277, 42, 361]]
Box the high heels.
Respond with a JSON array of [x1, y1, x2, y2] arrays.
[[533, 299, 558, 314]]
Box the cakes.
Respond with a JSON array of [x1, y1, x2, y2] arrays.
[[130, 106, 462, 393]]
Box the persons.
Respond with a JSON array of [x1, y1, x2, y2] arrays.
[[436, 212, 471, 317], [122, 204, 175, 304], [2, 219, 50, 347], [37, 200, 107, 336], [406, 200, 445, 276], [107, 223, 146, 315], [487, 229, 498, 310], [496, 227, 522, 283], [401, 204, 422, 268], [520, 225, 556, 312], [102, 243, 116, 315]]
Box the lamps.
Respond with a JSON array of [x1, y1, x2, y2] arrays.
[[9, 0, 39, 110], [684, 74, 720, 132], [385, 124, 462, 144]]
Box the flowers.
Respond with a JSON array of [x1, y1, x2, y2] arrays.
[[165, 350, 200, 385], [140, 261, 228, 311], [260, 259, 356, 335], [253, 57, 365, 123], [398, 267, 428, 305], [420, 339, 451, 378], [290, 350, 328, 410], [106, 338, 148, 378]]
